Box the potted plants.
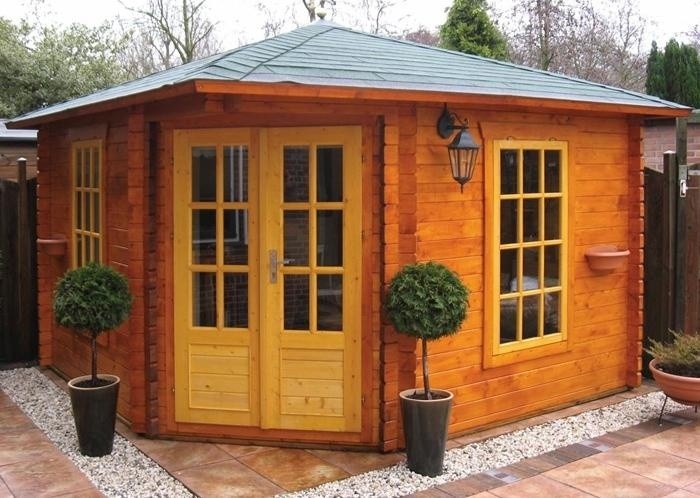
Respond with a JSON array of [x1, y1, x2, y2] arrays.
[[641, 328, 700, 426], [385, 259, 474, 478], [51, 259, 137, 457]]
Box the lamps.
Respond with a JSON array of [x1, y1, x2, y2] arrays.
[[433, 104, 481, 195]]
